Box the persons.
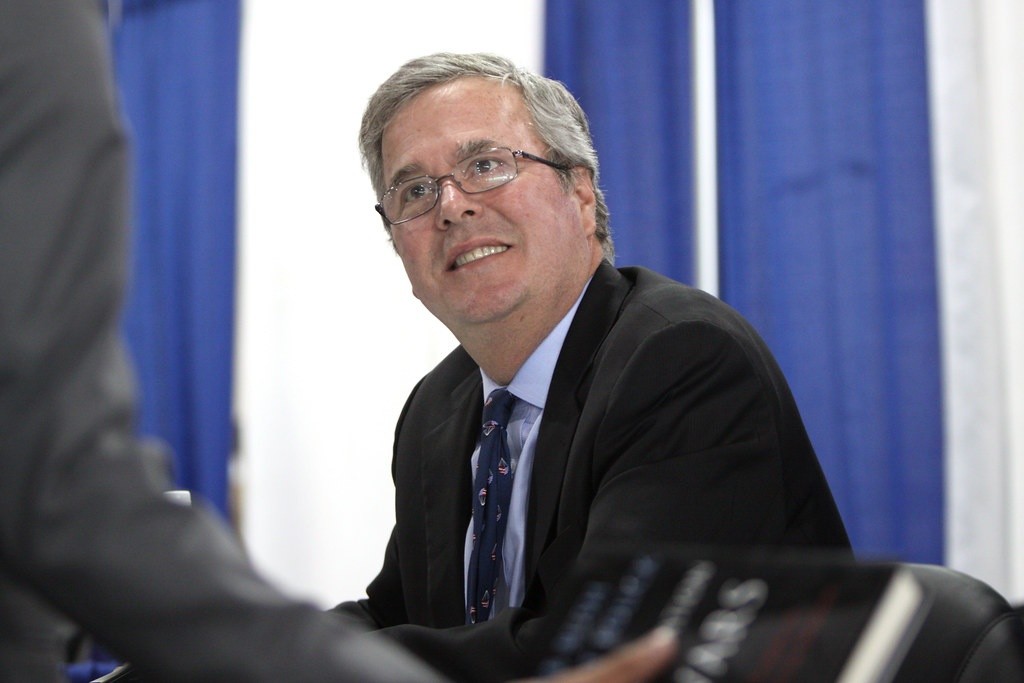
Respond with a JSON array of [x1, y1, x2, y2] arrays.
[[0, 0, 682, 683], [322, 52, 854, 683]]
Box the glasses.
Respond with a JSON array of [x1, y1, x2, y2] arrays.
[[375, 146, 571, 225]]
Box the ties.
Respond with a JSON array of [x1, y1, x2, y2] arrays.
[[466, 388, 518, 626]]
